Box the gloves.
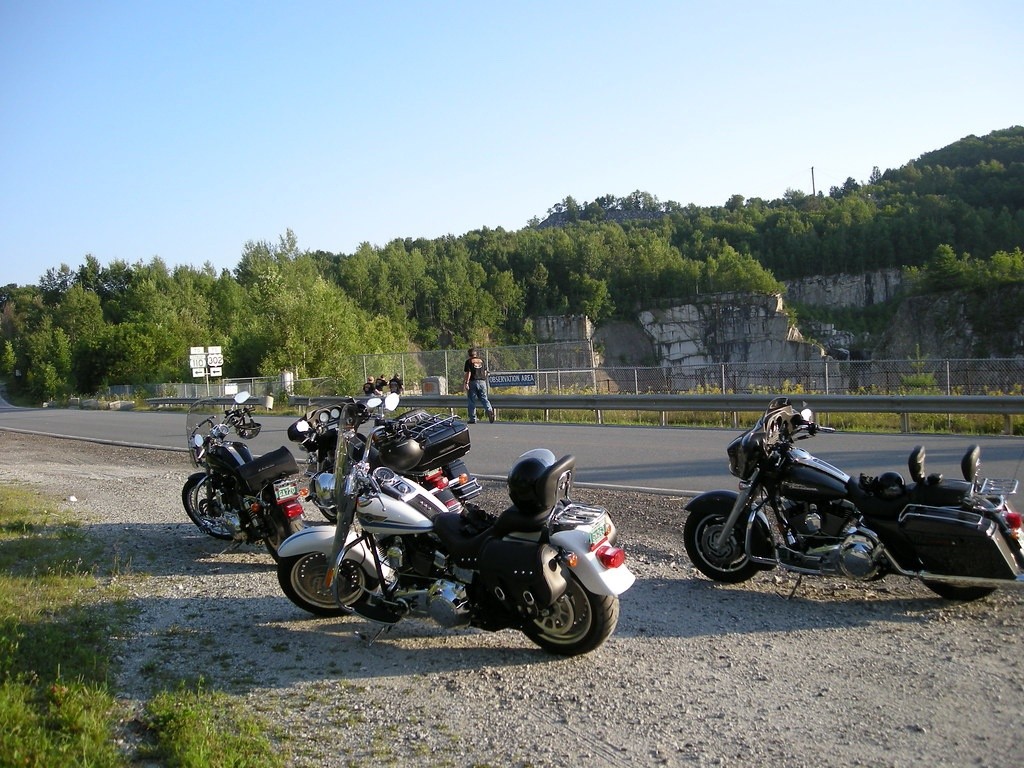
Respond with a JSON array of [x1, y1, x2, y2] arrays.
[[858, 470, 883, 496]]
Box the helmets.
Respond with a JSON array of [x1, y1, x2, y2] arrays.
[[873, 471, 907, 500], [377, 436, 426, 475], [234, 421, 262, 440], [467, 348, 480, 358]]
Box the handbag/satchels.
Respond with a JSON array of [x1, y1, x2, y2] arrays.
[[235, 446, 301, 494]]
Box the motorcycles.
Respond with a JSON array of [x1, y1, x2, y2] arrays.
[[181, 390, 312, 565], [683, 397, 1021, 603], [276, 390, 636, 657], [289, 396, 484, 520]]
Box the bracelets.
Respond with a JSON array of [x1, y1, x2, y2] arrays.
[[465, 382, 469, 386]]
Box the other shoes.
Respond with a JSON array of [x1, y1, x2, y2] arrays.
[[489, 412, 496, 423], [466, 419, 476, 424]]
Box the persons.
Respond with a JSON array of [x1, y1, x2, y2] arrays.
[[375, 374, 389, 396], [464, 348, 495, 424], [362, 376, 381, 396], [388, 373, 404, 395]]
